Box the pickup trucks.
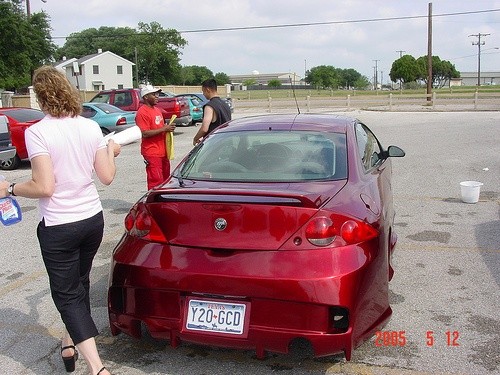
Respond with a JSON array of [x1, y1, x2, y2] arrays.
[[89, 88, 192, 128], [0, 115, 17, 161]]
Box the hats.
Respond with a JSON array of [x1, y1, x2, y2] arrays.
[[141, 85, 163, 97]]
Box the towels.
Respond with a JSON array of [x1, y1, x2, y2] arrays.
[[166, 114, 178, 161]]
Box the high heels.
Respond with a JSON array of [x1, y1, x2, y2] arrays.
[[60, 345, 78, 372]]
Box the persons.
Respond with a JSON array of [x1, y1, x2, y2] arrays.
[[136, 85, 176, 191], [193, 79, 231, 146], [0, 66, 122, 375]]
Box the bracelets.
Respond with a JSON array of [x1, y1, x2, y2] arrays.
[[12, 183, 16, 196]]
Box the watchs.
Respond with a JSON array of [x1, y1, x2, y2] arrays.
[[8, 185, 12, 194]]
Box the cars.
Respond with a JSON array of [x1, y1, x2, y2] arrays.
[[175, 93, 210, 106], [105, 110, 407, 364], [188, 97, 203, 122], [0, 107, 47, 171], [80, 102, 138, 137]]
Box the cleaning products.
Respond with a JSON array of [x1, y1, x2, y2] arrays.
[[0, 174, 23, 227]]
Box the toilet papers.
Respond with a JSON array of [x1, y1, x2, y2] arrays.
[[103, 124, 143, 146]]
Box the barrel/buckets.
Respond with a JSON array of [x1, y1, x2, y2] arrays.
[[460, 181, 484, 203]]
